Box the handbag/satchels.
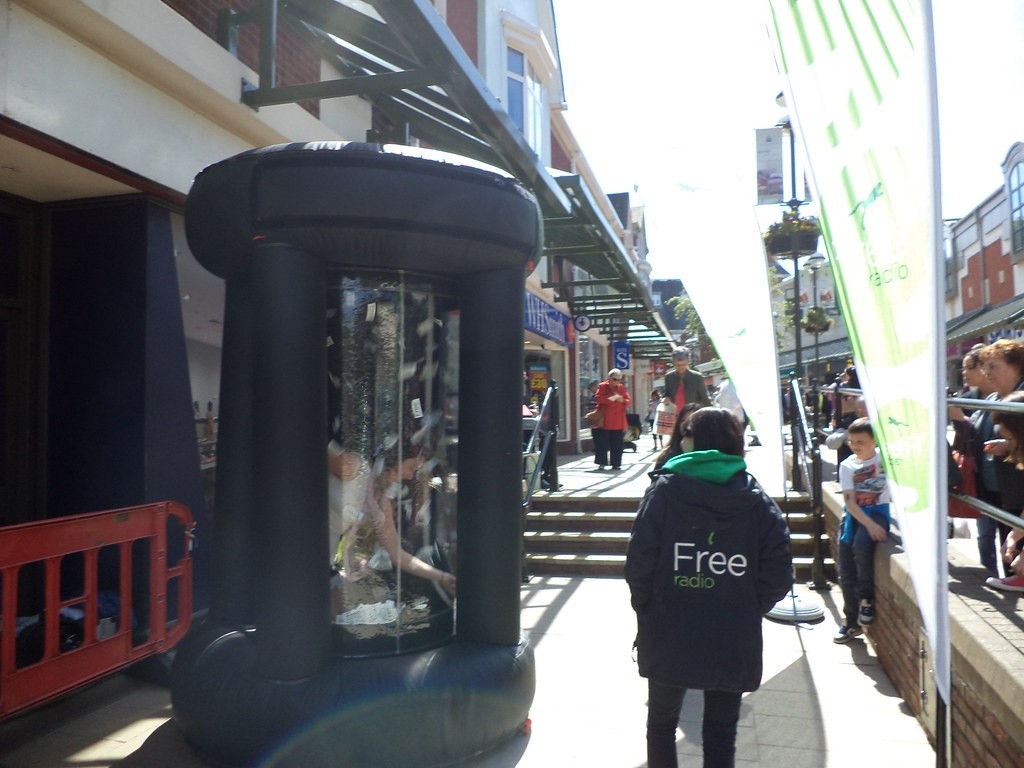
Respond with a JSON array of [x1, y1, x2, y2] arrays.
[[946, 416, 982, 519], [651, 398, 677, 436], [585, 411, 604, 429]]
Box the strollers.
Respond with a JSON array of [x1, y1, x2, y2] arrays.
[[621, 413, 643, 454]]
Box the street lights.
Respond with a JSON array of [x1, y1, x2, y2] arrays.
[[773, 90, 811, 399], [803, 250, 827, 387]]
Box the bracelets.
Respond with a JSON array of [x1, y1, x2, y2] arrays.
[[438, 571, 445, 583]]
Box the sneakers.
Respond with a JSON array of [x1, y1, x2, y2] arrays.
[[986, 572, 1024, 592], [833, 624, 863, 644], [857, 604, 875, 627]]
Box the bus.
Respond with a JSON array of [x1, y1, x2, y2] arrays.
[[778, 350, 856, 413]]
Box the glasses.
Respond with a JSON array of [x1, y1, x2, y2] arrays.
[[680, 428, 694, 438], [610, 377, 622, 382]]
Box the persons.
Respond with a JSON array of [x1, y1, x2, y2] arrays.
[[590, 369, 631, 470], [949, 341, 1023, 591], [329, 418, 454, 593], [645, 346, 754, 453], [655, 403, 702, 470], [625, 408, 793, 768], [833, 417, 891, 643], [781, 365, 863, 427]]
